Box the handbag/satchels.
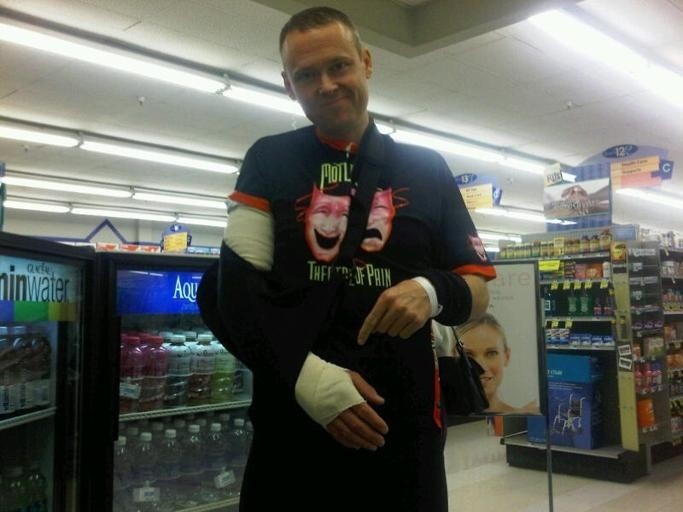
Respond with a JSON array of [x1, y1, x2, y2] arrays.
[[437, 354, 490, 417]]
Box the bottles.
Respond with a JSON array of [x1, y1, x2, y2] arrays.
[[113, 323, 253, 512], [0, 324, 52, 512]]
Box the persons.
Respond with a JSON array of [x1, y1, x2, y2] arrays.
[[217, 7, 497, 512], [545, 184, 610, 218], [456, 312, 541, 437]]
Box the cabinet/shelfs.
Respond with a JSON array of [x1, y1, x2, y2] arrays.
[[493, 244, 683, 482]]
